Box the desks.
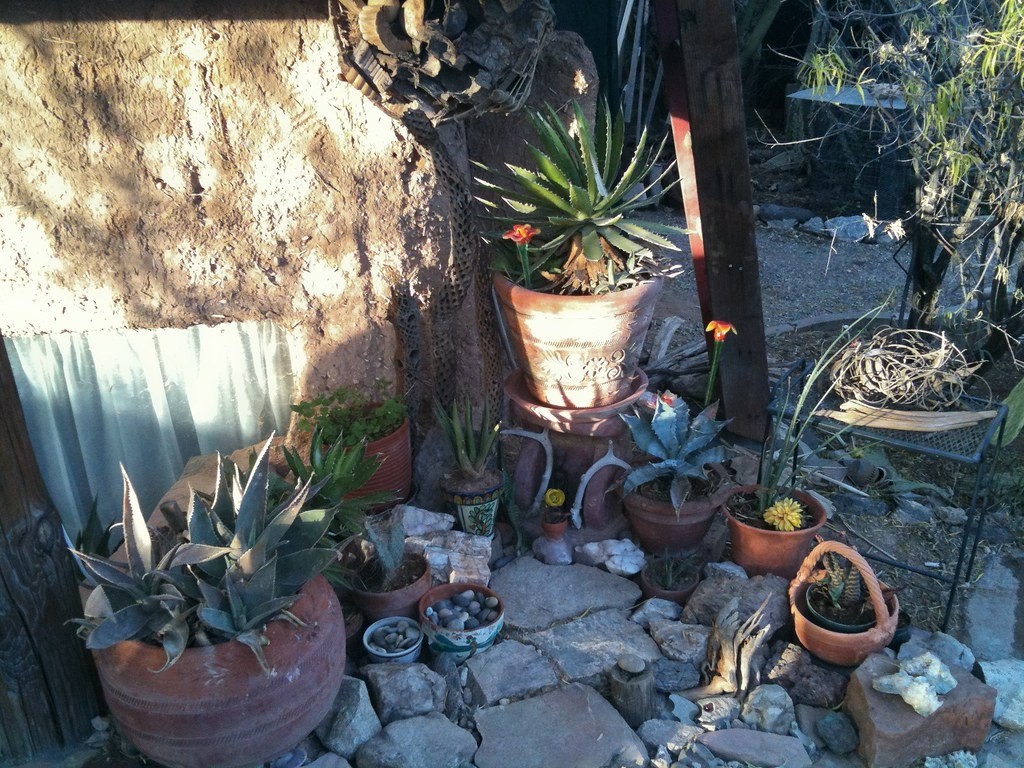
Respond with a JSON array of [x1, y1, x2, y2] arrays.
[[759, 362, 1010, 634]]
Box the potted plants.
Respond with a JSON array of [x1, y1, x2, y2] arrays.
[[430, 393, 507, 536], [62, 430, 348, 768], [349, 519, 433, 620], [643, 547, 703, 604], [243, 425, 404, 659], [289, 377, 413, 514], [787, 534, 900, 670]]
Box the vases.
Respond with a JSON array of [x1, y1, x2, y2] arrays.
[[540, 515, 568, 542], [721, 482, 828, 577], [362, 616, 425, 664], [613, 454, 734, 556], [485, 268, 669, 409], [498, 362, 647, 434], [419, 581, 507, 660]]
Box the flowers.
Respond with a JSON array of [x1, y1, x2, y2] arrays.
[[752, 286, 898, 531], [472, 92, 701, 293], [543, 487, 567, 522], [612, 318, 760, 526]]
